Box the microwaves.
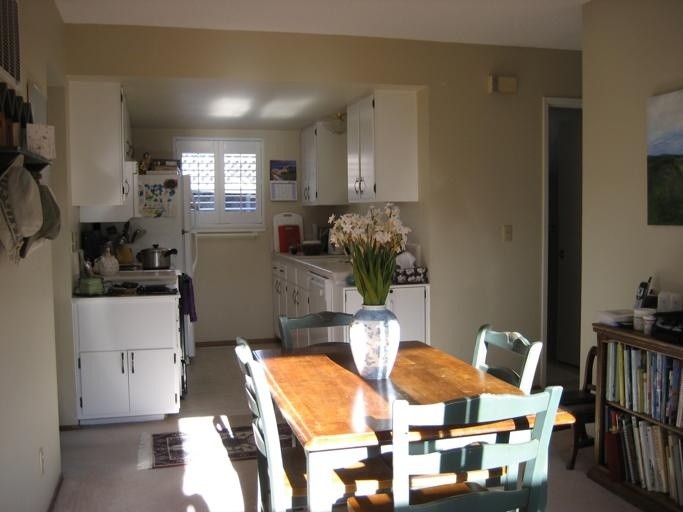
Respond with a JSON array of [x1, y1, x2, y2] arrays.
[[76, 160, 147, 224]]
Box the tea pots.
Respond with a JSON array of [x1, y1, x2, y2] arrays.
[[93, 254, 120, 277]]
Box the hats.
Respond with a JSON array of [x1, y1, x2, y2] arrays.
[[19, 184, 62, 260], [0, 166, 44, 260]]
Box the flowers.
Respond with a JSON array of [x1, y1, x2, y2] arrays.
[[328, 201, 413, 305]]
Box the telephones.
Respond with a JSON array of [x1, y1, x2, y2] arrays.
[[632, 276, 652, 310]]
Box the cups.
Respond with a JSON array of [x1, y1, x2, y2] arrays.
[[641, 315, 656, 337], [114, 243, 135, 265]]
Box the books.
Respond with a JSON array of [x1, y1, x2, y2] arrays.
[[596, 309, 633, 327], [601, 341, 683, 509]]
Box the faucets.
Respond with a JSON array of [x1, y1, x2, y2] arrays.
[[345, 258, 353, 265]]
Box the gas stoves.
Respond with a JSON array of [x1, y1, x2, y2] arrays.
[[72, 281, 179, 297]]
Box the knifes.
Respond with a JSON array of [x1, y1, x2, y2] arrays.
[[120, 220, 141, 243]]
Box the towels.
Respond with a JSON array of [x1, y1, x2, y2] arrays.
[[178, 272, 198, 321]]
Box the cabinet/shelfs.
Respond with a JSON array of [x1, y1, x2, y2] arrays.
[[586, 323, 683, 512], [300, 122, 347, 206], [271, 259, 431, 351], [72, 294, 180, 426], [79, 159, 139, 223], [347, 91, 419, 202], [67, 82, 134, 206]]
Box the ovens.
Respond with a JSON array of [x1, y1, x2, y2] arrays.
[[175, 298, 187, 413]]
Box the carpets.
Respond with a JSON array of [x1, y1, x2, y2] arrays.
[[137, 422, 303, 471]]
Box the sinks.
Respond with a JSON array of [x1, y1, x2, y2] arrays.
[[307, 258, 354, 275]]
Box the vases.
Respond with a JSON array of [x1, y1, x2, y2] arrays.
[[349, 305, 401, 381]]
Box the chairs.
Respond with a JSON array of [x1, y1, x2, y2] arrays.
[[345, 385, 564, 512], [277, 310, 355, 448], [472, 323, 543, 395], [564, 345, 597, 470], [233, 335, 393, 512]]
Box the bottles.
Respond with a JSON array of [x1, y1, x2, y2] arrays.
[[632, 307, 656, 331]]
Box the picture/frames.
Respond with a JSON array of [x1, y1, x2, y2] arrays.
[[269, 181, 297, 201]]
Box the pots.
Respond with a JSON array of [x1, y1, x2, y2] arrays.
[[300, 239, 323, 254], [136, 243, 178, 270]]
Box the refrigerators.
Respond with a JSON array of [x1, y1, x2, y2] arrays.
[[99, 173, 197, 366]]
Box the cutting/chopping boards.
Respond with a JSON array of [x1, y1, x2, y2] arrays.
[[272, 211, 304, 253]]
